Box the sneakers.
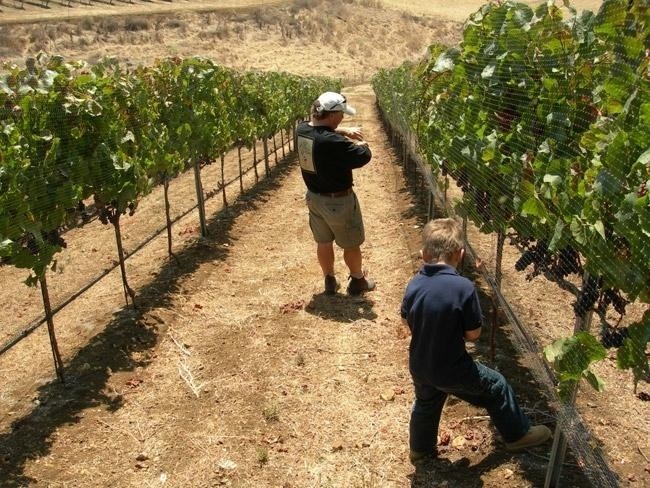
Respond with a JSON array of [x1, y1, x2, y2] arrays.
[[324, 276, 341, 294], [410, 451, 439, 464], [504, 425, 553, 451], [348, 275, 375, 294]]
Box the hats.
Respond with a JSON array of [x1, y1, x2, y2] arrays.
[[315, 90, 356, 115]]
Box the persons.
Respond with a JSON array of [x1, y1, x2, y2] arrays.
[[398, 217, 551, 464], [295, 90, 378, 294]]
